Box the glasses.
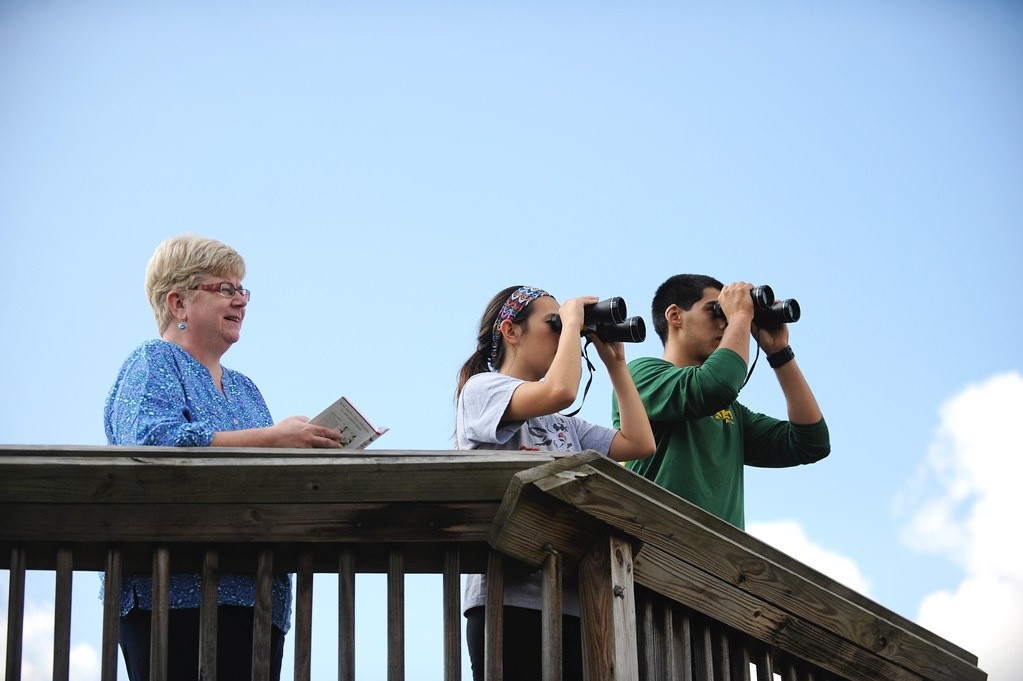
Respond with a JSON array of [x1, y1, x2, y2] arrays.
[[177, 282, 250, 301]]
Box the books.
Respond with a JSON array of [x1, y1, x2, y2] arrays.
[[309, 396, 390, 450]]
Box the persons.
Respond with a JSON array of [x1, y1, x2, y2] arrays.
[[98, 236, 344, 681], [611, 274, 832, 533], [447, 285, 656, 681]]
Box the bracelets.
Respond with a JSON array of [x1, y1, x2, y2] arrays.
[[768, 345, 795, 369]]
